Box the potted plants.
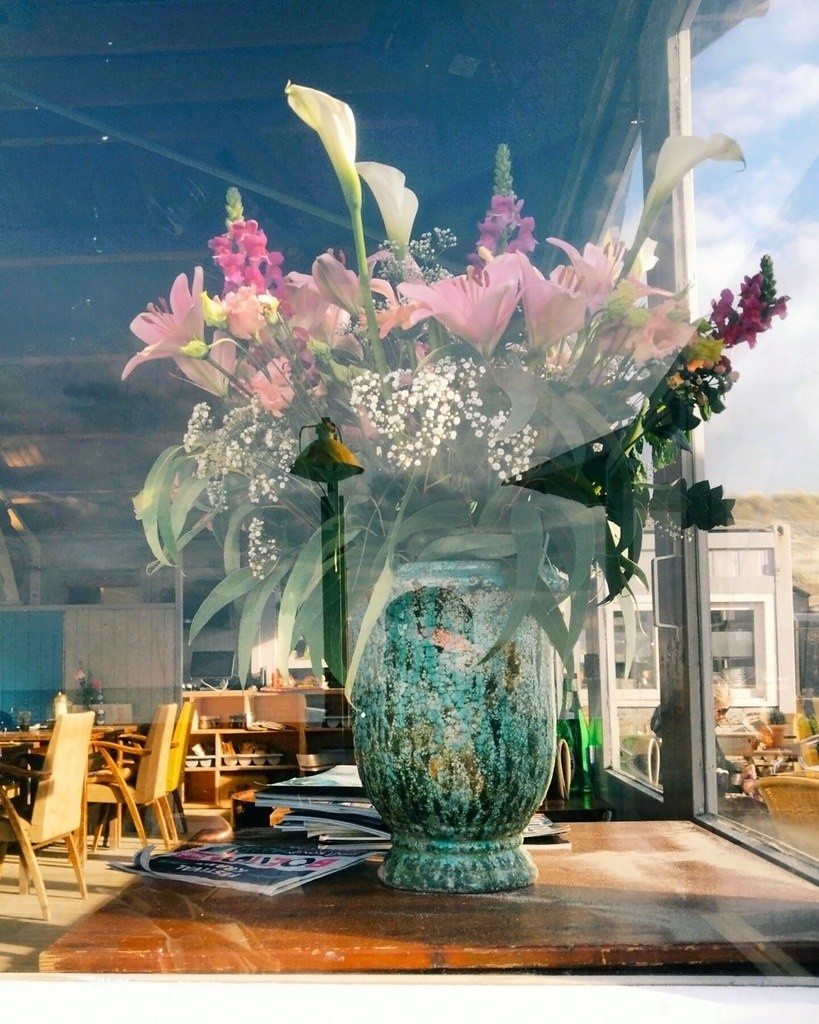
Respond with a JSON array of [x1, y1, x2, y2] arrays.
[[767, 709, 787, 748]]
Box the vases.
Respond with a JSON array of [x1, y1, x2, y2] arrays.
[[346, 555, 560, 895]]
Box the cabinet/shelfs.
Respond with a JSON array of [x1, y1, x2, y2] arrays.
[[178, 691, 355, 810]]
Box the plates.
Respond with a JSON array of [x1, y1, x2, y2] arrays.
[[712, 666, 755, 688]]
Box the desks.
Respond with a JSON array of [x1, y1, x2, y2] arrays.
[[747, 748, 794, 779], [38, 820, 819, 989], [537, 794, 618, 822]]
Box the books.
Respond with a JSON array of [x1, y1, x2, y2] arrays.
[[108, 764, 573, 896]]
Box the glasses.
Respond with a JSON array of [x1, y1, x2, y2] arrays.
[[717, 707, 730, 717]]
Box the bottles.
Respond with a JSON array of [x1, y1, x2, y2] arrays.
[[558, 673, 592, 794]]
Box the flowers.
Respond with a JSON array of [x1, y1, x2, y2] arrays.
[[72, 668, 103, 703], [116, 78, 792, 687]]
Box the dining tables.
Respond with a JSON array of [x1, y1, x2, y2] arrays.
[[0, 724, 141, 870]]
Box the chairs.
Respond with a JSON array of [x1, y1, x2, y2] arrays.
[[0, 699, 196, 921], [753, 777, 819, 864]]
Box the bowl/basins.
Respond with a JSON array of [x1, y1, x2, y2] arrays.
[[225, 759, 237, 766], [267, 757, 280, 764], [239, 758, 252, 766], [328, 720, 339, 728], [184, 760, 199, 767], [200, 760, 213, 767], [253, 757, 265, 765]]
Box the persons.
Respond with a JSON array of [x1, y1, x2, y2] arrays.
[[650, 677, 777, 836], [287, 639, 311, 660]]
